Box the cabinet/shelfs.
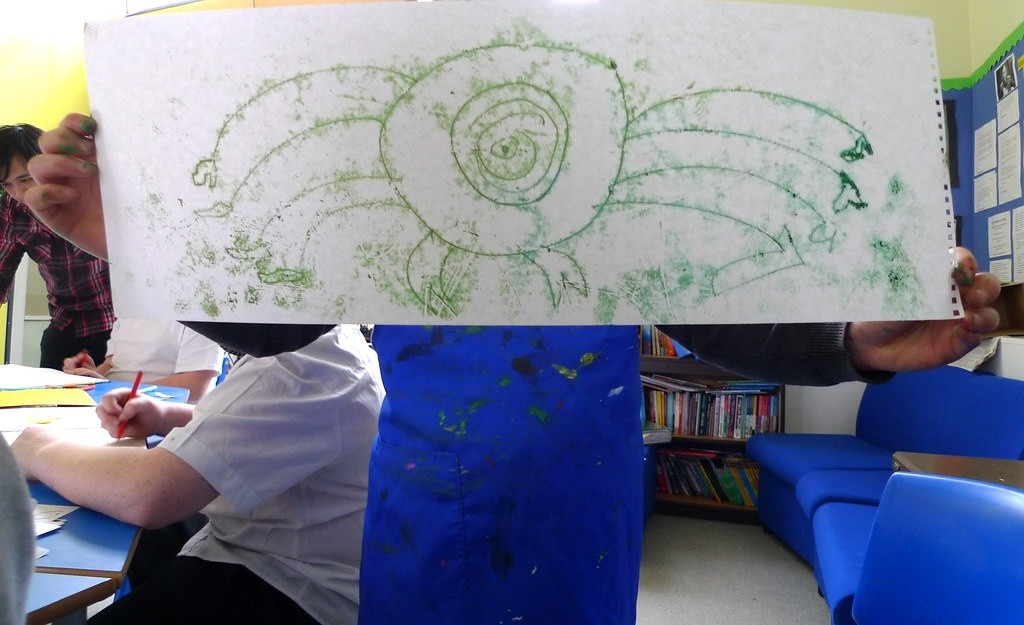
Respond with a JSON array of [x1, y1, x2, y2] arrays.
[[644, 325, 788, 529]]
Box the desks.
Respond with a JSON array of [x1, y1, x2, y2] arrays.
[[0, 381, 193, 625]]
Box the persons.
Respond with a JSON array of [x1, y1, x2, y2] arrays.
[[0, 325, 390, 625], [0, 123, 118, 372], [23, 112, 1001, 625], [65, 318, 224, 406]]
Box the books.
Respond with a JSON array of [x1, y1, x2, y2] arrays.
[[638, 324, 779, 506]]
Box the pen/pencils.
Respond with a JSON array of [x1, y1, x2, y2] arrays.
[[63, 384, 96, 391], [79, 349, 87, 368], [117, 370, 144, 443]]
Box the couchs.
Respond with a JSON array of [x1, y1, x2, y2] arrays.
[[744, 364, 1024, 625]]
